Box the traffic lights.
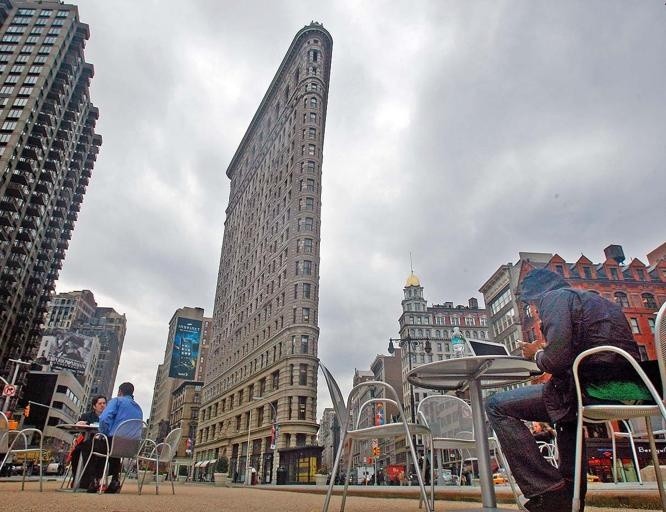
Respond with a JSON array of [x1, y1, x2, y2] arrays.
[[373, 447, 376, 456], [22, 405, 30, 418], [377, 448, 380, 456]]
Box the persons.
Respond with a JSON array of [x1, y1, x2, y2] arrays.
[[83, 381, 143, 493], [70, 395, 107, 489], [483, 267, 642, 512], [462, 471, 471, 486]]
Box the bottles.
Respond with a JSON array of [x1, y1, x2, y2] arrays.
[[450, 327, 464, 360]]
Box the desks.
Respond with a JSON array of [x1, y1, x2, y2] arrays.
[[55, 424, 99, 493], [405, 355, 544, 510]]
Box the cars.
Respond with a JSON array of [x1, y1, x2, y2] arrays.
[[490, 473, 509, 486], [586, 473, 600, 484], [338, 463, 461, 488], [2, 463, 46, 476]]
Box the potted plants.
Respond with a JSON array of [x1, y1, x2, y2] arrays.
[[179, 467, 188, 484], [213, 456, 229, 487], [314, 468, 329, 485]]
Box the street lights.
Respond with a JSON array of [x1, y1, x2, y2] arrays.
[[388, 328, 432, 486], [0, 358, 32, 422], [251, 395, 279, 486]]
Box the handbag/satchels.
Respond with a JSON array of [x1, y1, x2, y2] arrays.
[[578, 360, 661, 406]]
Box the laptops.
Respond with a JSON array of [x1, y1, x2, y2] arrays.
[[465, 338, 521, 357]]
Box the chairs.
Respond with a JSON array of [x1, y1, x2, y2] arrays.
[[318, 356, 430, 512], [571, 302, 666, 510], [0, 411, 47, 492], [416, 395, 524, 510], [73, 419, 183, 495]]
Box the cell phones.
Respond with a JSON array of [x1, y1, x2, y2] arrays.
[[181, 338, 193, 364]]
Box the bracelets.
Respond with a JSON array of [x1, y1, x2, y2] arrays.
[[534, 347, 543, 362]]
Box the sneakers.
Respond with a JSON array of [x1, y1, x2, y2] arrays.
[[71, 481, 121, 495], [518, 484, 584, 512]]
